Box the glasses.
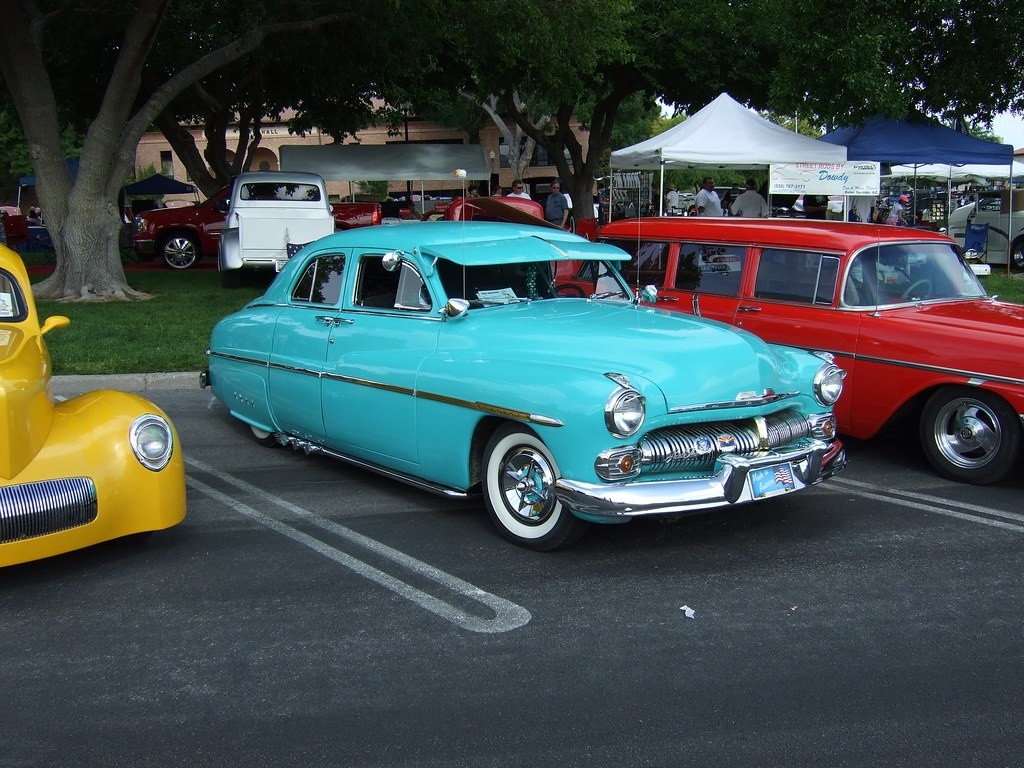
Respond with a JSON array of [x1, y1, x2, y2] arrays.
[[516, 187, 523, 189], [553, 187, 560, 189]]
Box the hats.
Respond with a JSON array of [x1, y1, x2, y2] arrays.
[[900, 195, 909, 204]]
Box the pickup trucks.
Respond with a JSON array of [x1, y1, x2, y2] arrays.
[[217, 171, 337, 289], [132, 183, 382, 270]]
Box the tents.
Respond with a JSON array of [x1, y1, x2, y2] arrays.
[[886, 162, 1024, 236], [279, 144, 491, 215], [122, 173, 201, 223], [814, 104, 1015, 277], [608, 92, 848, 223], [16, 156, 79, 216]]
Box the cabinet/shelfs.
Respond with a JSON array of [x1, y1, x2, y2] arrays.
[[870, 183, 1002, 228]]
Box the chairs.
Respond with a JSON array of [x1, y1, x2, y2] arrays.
[[961, 218, 989, 263], [253, 183, 271, 196]]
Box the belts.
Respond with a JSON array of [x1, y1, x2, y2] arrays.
[[550, 217, 562, 222]]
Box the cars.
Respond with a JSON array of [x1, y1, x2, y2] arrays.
[[562, 215, 1024, 484], [0, 243, 186, 569], [199, 219, 849, 554], [421, 196, 596, 298], [948, 197, 1024, 272]]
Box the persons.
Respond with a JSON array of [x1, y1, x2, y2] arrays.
[[802, 195, 829, 220], [28, 202, 41, 219], [491, 186, 502, 197], [848, 196, 876, 224], [721, 183, 742, 216], [886, 194, 910, 226], [653, 187, 666, 219], [664, 182, 679, 216], [730, 178, 768, 218], [544, 180, 569, 228], [155, 194, 163, 209], [507, 179, 531, 200], [694, 176, 723, 217], [467, 185, 480, 198]]
